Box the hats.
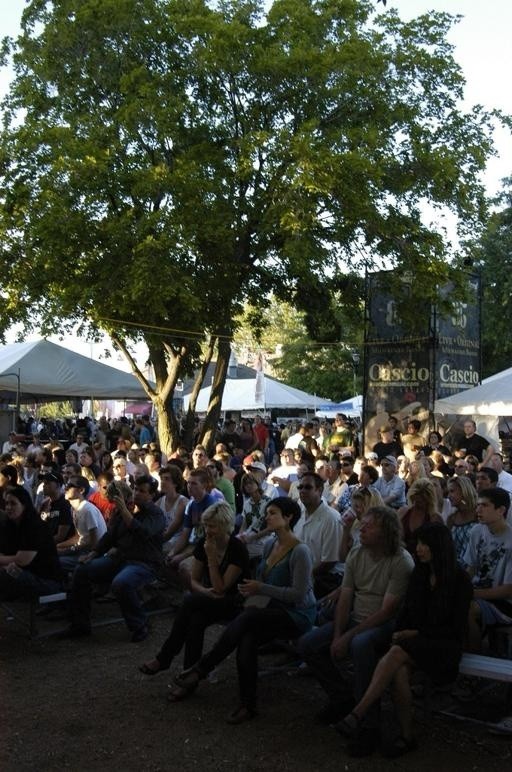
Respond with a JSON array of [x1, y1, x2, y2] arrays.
[[379, 423, 394, 434], [366, 452, 378, 460], [315, 459, 328, 470], [37, 472, 65, 484], [337, 449, 352, 458], [380, 454, 398, 469], [242, 454, 260, 466], [245, 461, 268, 475], [49, 432, 59, 440]]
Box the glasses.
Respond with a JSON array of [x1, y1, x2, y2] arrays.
[[65, 482, 85, 491], [112, 463, 127, 470], [466, 460, 476, 466], [138, 454, 146, 457], [339, 463, 353, 468], [453, 464, 466, 468], [336, 418, 346, 421], [324, 463, 339, 470], [298, 484, 320, 490], [279, 453, 294, 457], [206, 464, 219, 469], [193, 453, 206, 457]]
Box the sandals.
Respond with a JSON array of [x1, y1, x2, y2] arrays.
[[223, 702, 260, 725], [137, 654, 172, 677], [380, 737, 419, 760], [173, 666, 208, 688], [167, 687, 196, 703], [333, 711, 367, 737]]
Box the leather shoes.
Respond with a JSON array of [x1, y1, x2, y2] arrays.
[[47, 612, 66, 622], [55, 625, 91, 638], [131, 624, 148, 642], [35, 605, 50, 615]]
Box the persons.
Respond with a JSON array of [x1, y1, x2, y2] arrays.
[[0, 412, 512, 757]]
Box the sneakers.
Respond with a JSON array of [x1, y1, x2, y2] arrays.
[[347, 727, 383, 758], [316, 704, 348, 724]]
